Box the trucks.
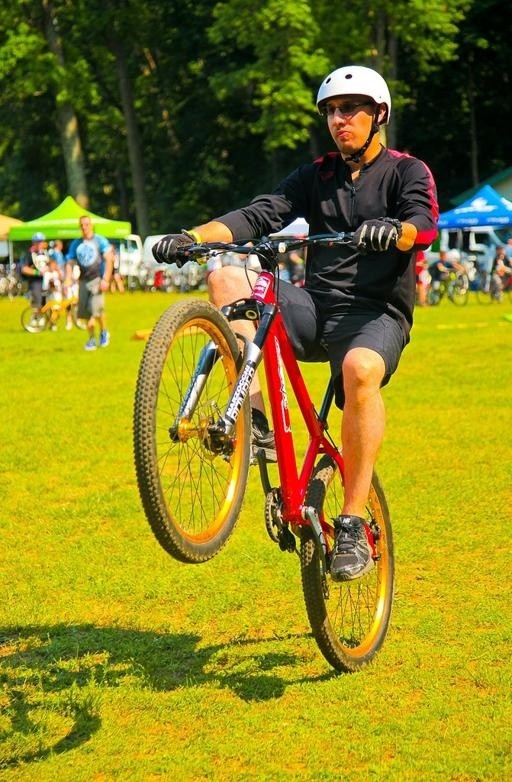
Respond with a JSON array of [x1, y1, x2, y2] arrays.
[[425, 224, 511, 292], [120, 235, 166, 258]]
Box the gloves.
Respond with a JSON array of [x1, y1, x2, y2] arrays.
[[151, 228, 196, 267], [351, 216, 403, 256]]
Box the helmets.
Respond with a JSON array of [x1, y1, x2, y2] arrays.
[[316, 65, 391, 126]]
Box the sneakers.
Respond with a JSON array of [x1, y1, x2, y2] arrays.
[[85, 339, 96, 350], [201, 412, 275, 451], [100, 331, 108, 346], [330, 515, 375, 581]]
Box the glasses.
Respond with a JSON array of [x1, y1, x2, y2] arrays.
[[319, 102, 369, 117]]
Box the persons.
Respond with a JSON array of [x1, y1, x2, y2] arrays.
[[429, 248, 460, 302], [211, 233, 305, 288], [22, 215, 126, 351], [489, 243, 510, 302], [149, 63, 440, 587], [414, 249, 431, 308]]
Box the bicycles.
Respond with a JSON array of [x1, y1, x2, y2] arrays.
[[475, 266, 511, 306], [425, 269, 469, 306], [21, 277, 97, 333], [120, 258, 202, 293], [0, 262, 32, 301], [133, 230, 396, 673]]
